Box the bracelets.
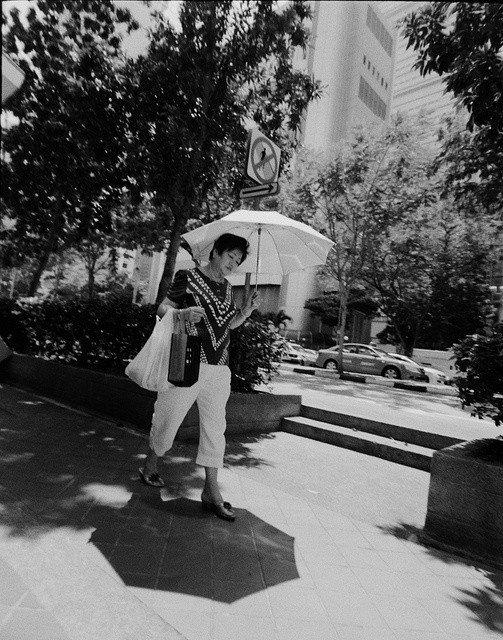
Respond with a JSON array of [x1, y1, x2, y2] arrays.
[[240, 305, 251, 318]]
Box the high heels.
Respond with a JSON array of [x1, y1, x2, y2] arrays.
[[201, 500, 237, 522], [138, 467, 164, 487]]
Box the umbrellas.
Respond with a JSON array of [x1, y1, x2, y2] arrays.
[[179, 209, 336, 289]]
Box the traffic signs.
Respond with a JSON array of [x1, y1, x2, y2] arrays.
[[239, 127, 281, 199]]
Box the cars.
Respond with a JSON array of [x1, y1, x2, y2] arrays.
[[386, 352, 451, 385], [272, 338, 319, 366], [303, 347, 319, 357], [314, 342, 427, 382]]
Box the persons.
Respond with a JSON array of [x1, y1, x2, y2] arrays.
[[138, 232, 262, 522]]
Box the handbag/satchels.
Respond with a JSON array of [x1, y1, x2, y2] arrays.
[[167, 309, 201, 387]]
